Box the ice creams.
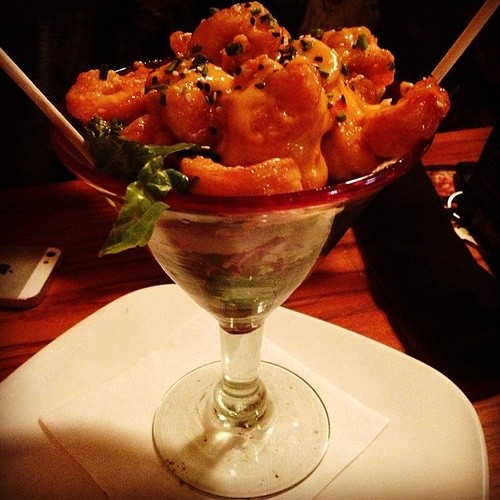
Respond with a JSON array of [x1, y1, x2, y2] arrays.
[[66, 3, 452, 196]]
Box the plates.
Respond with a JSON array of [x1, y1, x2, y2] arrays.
[[3, 283, 490, 500]]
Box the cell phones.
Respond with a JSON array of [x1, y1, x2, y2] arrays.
[[0, 242, 63, 308]]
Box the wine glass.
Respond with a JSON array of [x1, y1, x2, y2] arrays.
[[48, 143, 440, 499]]
